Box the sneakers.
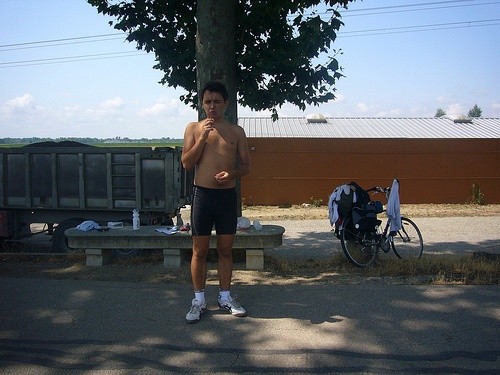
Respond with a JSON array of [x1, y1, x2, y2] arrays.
[[217, 295, 247, 316], [185, 298, 208, 322]]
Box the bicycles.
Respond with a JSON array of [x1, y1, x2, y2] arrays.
[[338, 186, 423, 268]]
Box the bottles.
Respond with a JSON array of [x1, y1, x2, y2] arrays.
[[132, 209, 141, 231]]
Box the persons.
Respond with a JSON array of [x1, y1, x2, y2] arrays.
[[181, 81, 246, 324]]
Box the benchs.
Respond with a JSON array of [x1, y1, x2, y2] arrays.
[[64, 225, 286, 271]]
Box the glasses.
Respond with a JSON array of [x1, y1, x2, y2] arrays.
[[95, 227, 111, 232]]
